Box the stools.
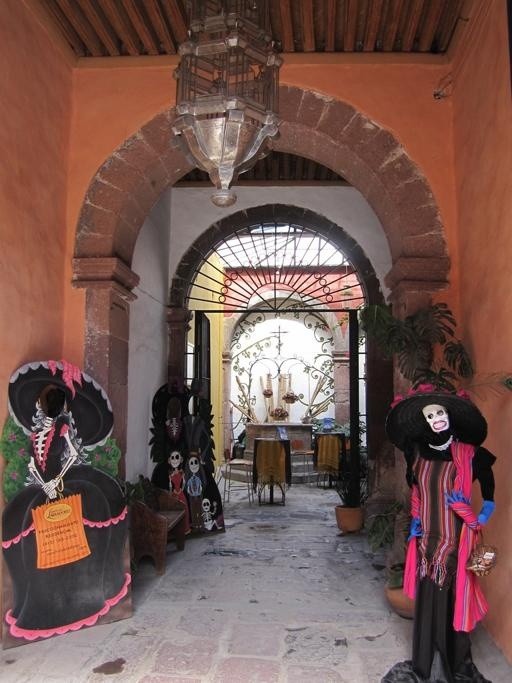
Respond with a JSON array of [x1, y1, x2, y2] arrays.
[[135, 489, 191, 572], [293, 450, 327, 488], [221, 456, 255, 505]]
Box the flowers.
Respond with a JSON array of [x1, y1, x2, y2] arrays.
[[269, 405, 289, 417], [283, 390, 298, 400]]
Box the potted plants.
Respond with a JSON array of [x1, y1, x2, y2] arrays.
[[327, 454, 373, 532]]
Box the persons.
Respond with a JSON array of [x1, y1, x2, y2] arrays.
[[405, 399, 495, 683], [0, 384, 130, 642]]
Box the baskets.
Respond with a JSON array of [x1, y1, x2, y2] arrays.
[[465, 523, 497, 576]]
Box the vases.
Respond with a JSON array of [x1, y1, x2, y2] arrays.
[[272, 415, 285, 420], [385, 557, 416, 620], [285, 399, 296, 403]]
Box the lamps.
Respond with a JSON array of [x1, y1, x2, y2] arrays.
[[167, 1, 282, 208]]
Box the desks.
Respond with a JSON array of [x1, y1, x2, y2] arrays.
[[254, 437, 290, 506], [314, 431, 347, 487]]
